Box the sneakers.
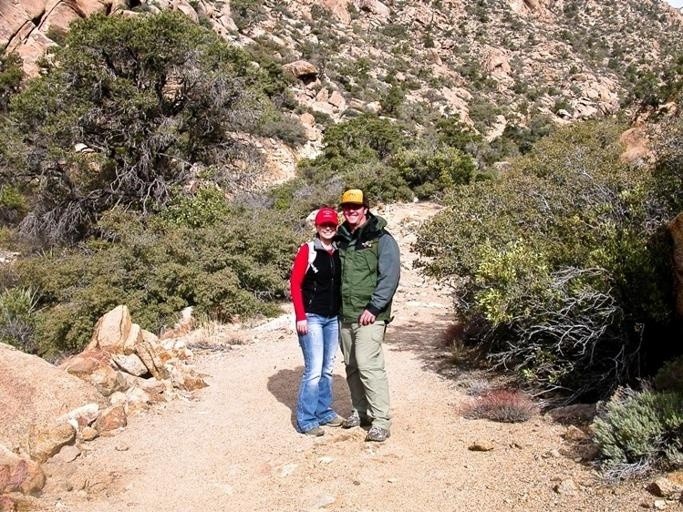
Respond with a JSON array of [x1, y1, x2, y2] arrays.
[[325, 412, 346, 427], [305, 425, 325, 436], [342, 411, 371, 429], [365, 425, 391, 442]]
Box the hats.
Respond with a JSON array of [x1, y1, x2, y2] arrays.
[[338, 188, 370, 209], [314, 207, 339, 227]]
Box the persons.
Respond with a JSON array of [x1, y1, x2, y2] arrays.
[[296, 189, 401, 442], [290, 208, 345, 436]]
